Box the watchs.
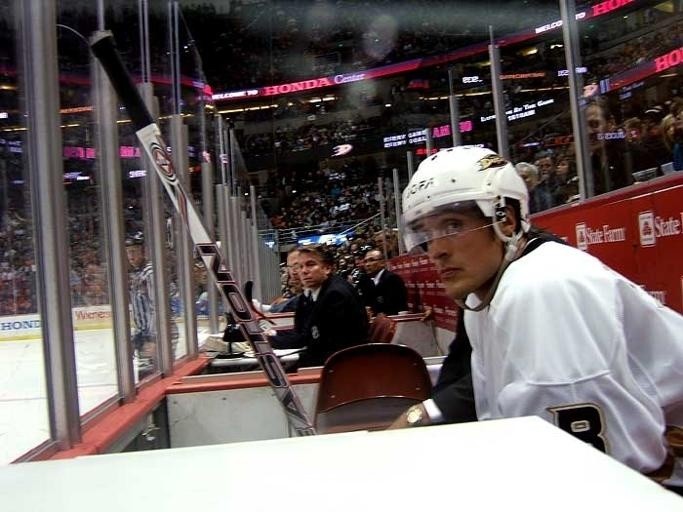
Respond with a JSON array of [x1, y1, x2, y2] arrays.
[[406, 405, 422, 427]]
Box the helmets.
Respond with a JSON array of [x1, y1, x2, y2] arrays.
[[123, 229, 148, 247], [400, 145, 532, 243]]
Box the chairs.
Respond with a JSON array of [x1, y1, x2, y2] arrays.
[[310, 312, 434, 422]]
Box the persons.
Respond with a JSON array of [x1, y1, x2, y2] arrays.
[[400, 145, 683, 488], [387, 307, 478, 431], [1, 22, 682, 377]]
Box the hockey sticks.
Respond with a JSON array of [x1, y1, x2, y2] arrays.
[[245, 281, 276, 325]]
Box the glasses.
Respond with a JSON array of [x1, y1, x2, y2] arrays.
[[363, 259, 381, 263], [284, 265, 299, 269]]
